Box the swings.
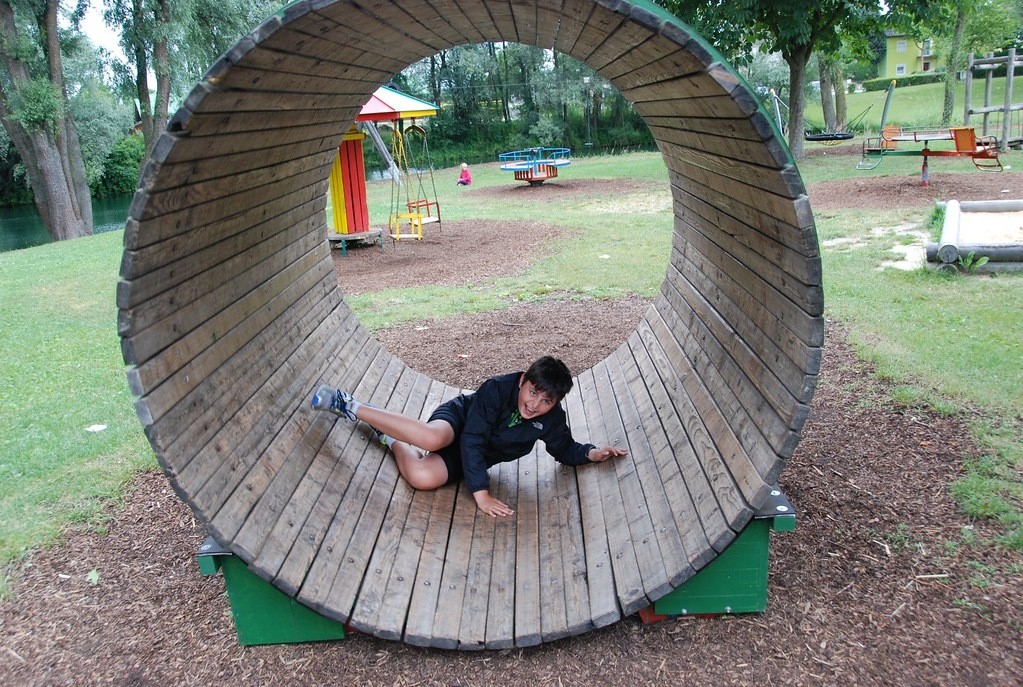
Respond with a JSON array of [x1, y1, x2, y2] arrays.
[[388, 126, 442, 240]]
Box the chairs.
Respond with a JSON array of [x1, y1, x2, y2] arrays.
[[856, 125, 902, 170], [950, 128, 1003, 173]]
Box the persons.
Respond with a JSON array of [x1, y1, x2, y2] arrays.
[[456, 163, 472, 185], [312, 356, 628, 517]]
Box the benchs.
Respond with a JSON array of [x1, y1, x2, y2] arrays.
[[499, 147, 571, 171]]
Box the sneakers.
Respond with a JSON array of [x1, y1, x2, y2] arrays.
[[362, 402, 387, 445], [310, 384, 358, 422]]
